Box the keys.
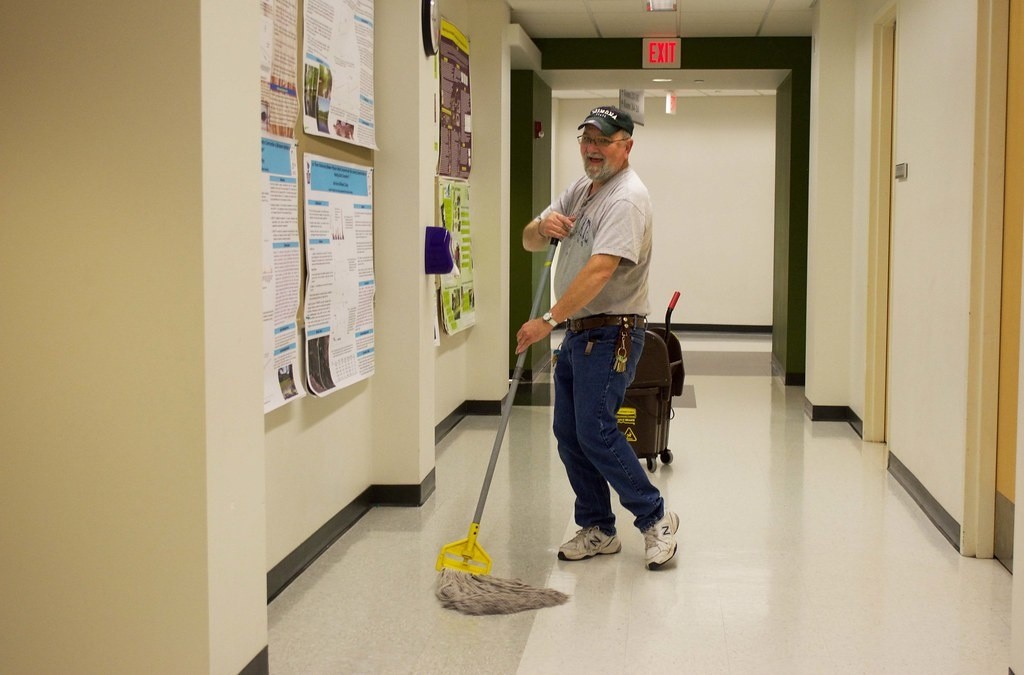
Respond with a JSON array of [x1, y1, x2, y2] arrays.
[[613, 355, 627, 372]]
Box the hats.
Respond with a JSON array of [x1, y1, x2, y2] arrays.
[[578, 105, 634, 136]]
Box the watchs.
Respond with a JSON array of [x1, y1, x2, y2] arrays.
[[542, 313, 558, 327]]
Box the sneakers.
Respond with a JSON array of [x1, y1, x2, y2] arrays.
[[558, 526, 622, 561], [642, 507, 679, 571]]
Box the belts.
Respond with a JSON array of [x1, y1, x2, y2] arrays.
[[566, 313, 645, 333]]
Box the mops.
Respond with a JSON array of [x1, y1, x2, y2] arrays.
[[435, 227, 569, 615]]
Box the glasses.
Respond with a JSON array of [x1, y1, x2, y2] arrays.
[[577, 135, 629, 147]]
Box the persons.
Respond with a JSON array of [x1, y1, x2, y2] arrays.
[[515, 107, 681, 571]]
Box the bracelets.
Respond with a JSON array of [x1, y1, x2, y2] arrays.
[[538, 221, 546, 237]]
